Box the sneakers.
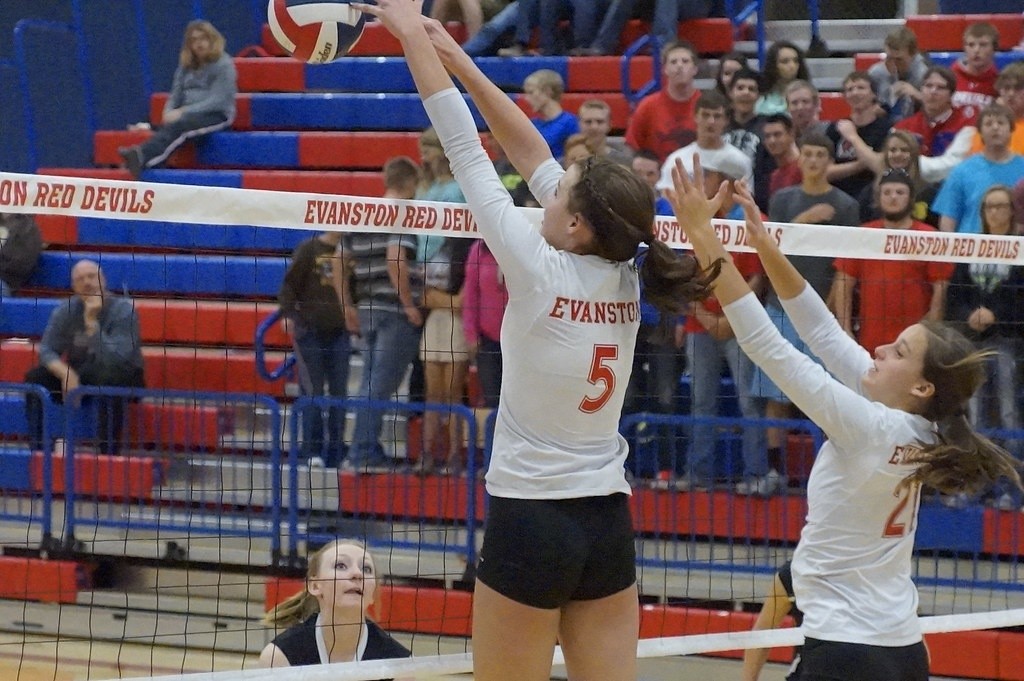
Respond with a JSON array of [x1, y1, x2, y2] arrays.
[[735, 471, 781, 496], [405, 450, 436, 476], [650, 477, 709, 493], [440, 451, 464, 475]]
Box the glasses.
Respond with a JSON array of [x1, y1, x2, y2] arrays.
[[983, 199, 1011, 212]]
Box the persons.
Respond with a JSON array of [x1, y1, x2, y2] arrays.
[[0, 212, 41, 297], [664, 153, 1024, 681], [260, 538, 415, 673], [25, 257, 142, 455], [431, 1, 729, 58], [352, 0, 729, 680], [275, 22, 1024, 510], [734, 557, 805, 681], [115, 19, 236, 177]]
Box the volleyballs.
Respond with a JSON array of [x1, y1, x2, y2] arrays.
[[267, 0, 366, 65]]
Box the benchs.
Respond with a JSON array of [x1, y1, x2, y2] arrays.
[[3, 14, 1023, 680]]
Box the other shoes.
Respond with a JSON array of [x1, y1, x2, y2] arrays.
[[118, 146, 143, 175], [340, 459, 397, 473], [306, 456, 325, 467]]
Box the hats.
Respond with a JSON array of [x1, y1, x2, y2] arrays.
[[878, 169, 916, 191]]
